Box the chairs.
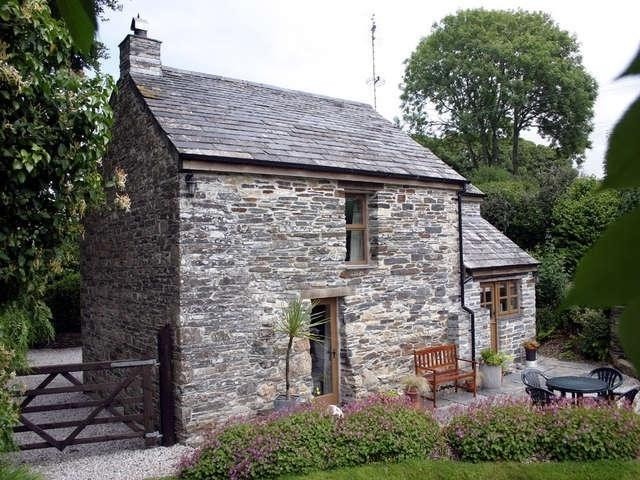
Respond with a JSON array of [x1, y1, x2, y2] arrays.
[[520, 366, 640, 411]]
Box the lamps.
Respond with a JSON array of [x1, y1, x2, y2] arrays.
[[535, 270, 540, 284], [186, 175, 201, 198]]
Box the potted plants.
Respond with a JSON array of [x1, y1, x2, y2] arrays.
[[402, 374, 430, 406], [521, 339, 540, 361], [479, 349, 515, 389], [274, 293, 333, 414]]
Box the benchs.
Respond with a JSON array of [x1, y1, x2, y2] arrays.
[[414, 344, 479, 408]]
